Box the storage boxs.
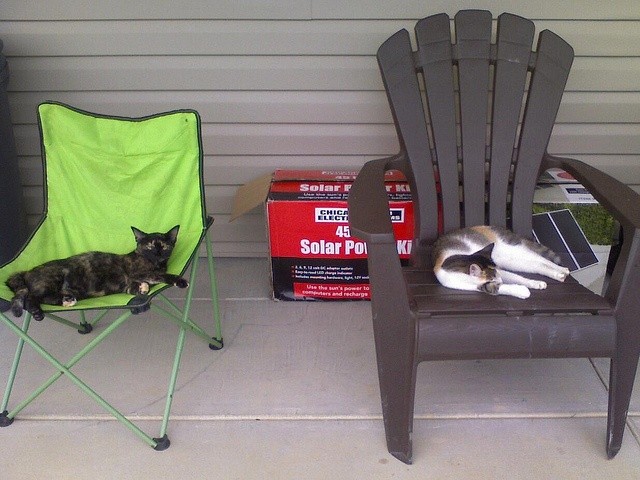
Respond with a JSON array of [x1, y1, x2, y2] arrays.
[[266, 170, 454, 303]]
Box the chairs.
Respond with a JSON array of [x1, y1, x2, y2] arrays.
[[0, 99, 221, 450], [348, 9, 640, 464]]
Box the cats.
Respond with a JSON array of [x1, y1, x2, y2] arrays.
[[434, 223, 571, 301]]
